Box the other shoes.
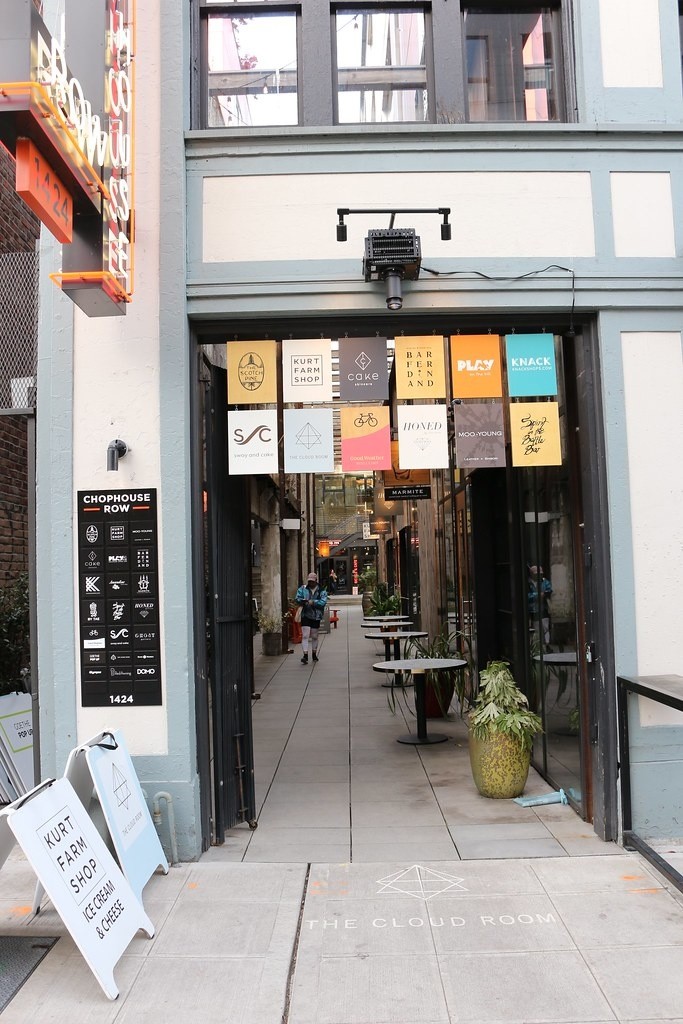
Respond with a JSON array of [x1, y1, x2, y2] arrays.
[[312, 654, 318, 661], [301, 657, 308, 664]]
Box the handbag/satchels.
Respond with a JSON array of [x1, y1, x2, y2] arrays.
[[294, 585, 305, 622], [300, 604, 322, 629]]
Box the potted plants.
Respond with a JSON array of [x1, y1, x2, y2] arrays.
[[468, 660, 547, 800], [368, 583, 401, 645], [385, 620, 479, 719], [358, 568, 381, 617]]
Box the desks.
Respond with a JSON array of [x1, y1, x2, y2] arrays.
[[364, 631, 429, 687], [372, 659, 468, 746], [361, 621, 413, 656], [533, 652, 580, 738], [363, 615, 409, 645]]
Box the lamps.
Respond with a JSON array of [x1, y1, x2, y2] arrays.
[[337, 206, 451, 311]]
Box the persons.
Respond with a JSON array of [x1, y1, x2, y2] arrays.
[[329, 569, 338, 596], [527, 565, 553, 653], [294, 573, 327, 664]]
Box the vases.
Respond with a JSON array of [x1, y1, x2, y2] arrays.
[[263, 632, 281, 657]]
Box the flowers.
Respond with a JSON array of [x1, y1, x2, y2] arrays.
[[254, 611, 294, 632]]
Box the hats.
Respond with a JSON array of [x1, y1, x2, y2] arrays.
[[308, 572, 317, 581]]
